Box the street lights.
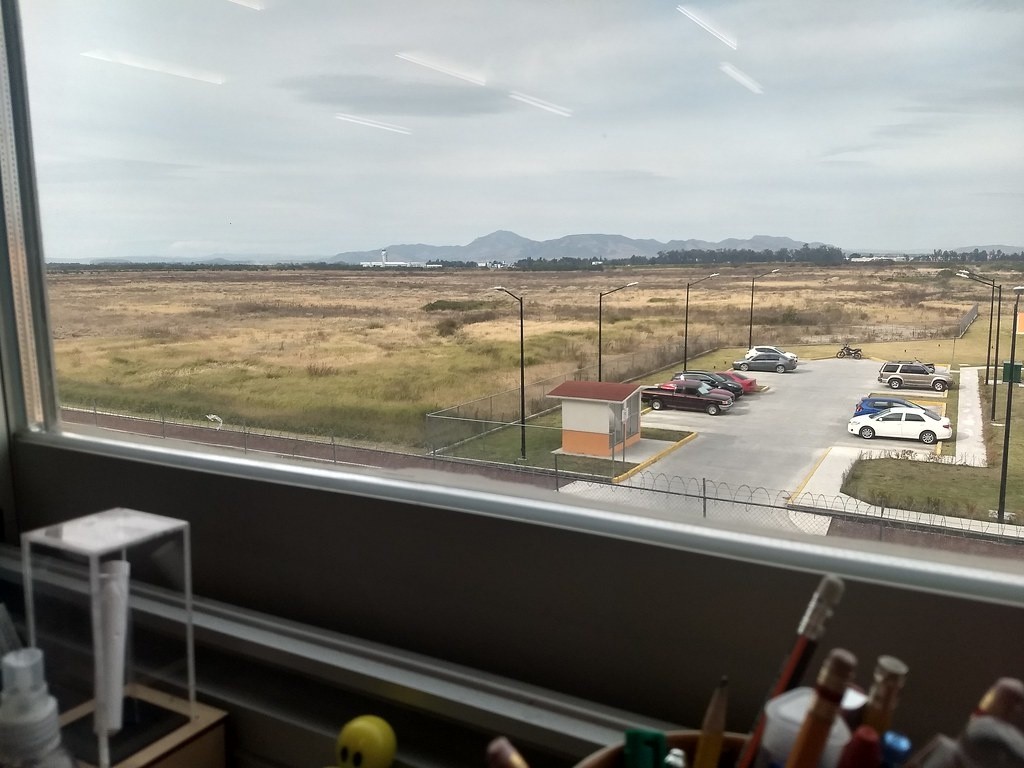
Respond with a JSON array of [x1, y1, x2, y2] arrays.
[[956, 273, 1001, 421], [494, 287, 526, 459], [599, 282, 638, 382], [749, 269, 779, 350], [998, 287, 1024, 524], [959, 270, 995, 385], [683, 273, 720, 371]]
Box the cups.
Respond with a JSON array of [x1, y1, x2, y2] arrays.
[[574, 732, 772, 768]]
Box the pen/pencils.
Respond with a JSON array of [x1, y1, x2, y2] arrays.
[[863, 654, 911, 729], [784, 647, 859, 768], [837, 724, 911, 768], [960, 677, 1024, 729], [487, 737, 528, 768], [693, 674, 729, 768], [735, 572, 846, 768]]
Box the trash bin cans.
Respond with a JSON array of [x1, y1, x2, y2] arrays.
[[1003, 361, 1023, 383]]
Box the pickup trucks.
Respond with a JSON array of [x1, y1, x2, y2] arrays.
[[641, 380, 733, 415]]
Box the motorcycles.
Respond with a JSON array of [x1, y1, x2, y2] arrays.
[[837, 344, 861, 360]]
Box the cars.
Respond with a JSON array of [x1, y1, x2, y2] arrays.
[[745, 346, 798, 364], [715, 372, 757, 392], [877, 362, 953, 392], [853, 397, 921, 417], [671, 371, 744, 399], [847, 407, 952, 444], [732, 353, 797, 373], [658, 380, 735, 402]]
